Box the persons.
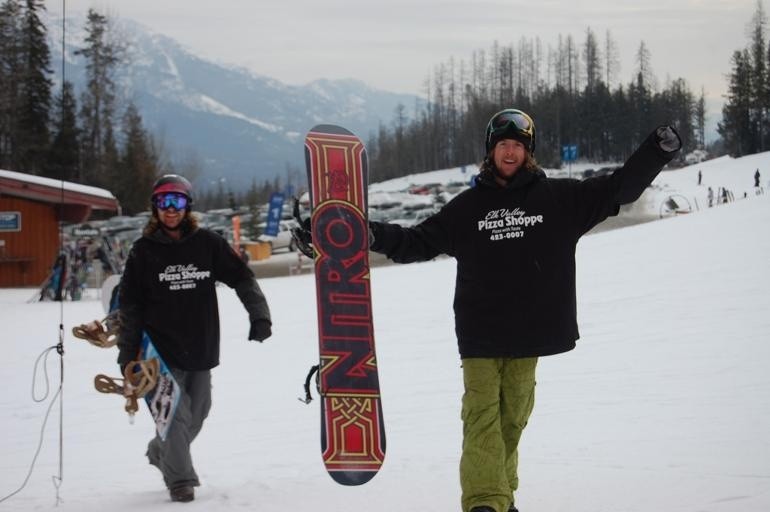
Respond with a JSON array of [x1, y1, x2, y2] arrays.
[[754, 168, 760, 186], [708, 187, 714, 207], [722, 188, 727, 203], [118, 173, 272, 501], [303, 109, 683, 512], [698, 171, 701, 184]]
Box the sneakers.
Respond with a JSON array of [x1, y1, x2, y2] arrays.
[[170, 485, 194, 501], [146, 439, 161, 470]]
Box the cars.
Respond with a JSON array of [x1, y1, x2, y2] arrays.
[[63, 197, 298, 253], [367, 180, 472, 221]]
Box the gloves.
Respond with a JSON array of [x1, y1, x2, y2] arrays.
[[248, 320, 272, 343], [120, 365, 137, 377], [655, 127, 680, 154]]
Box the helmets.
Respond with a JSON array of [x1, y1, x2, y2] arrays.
[[150, 174, 193, 203]]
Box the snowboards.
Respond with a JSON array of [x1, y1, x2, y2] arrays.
[[289, 125, 387, 487], [73, 275, 180, 443]]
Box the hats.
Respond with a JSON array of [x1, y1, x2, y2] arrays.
[[486, 109, 535, 158]]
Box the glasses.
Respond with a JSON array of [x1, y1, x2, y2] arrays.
[[490, 109, 534, 135], [153, 192, 189, 211]]
